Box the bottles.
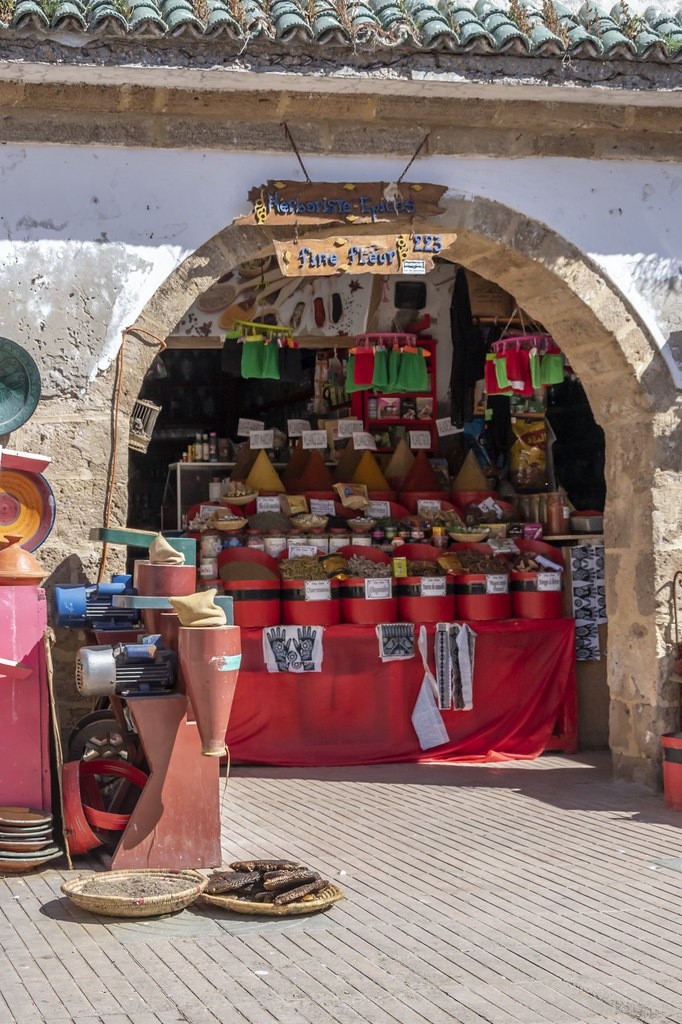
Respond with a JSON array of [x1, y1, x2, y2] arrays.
[[202, 434, 209, 462], [518, 492, 570, 535], [187, 445, 193, 462], [208, 478, 221, 502], [246, 528, 371, 559], [209, 433, 218, 462], [182, 514, 187, 529], [194, 433, 202, 462], [182, 452, 187, 462]]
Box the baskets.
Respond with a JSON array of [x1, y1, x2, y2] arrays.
[[200, 884, 343, 914], [60, 868, 209, 917]]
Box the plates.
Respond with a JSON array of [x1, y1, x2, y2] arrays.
[[0, 806, 65, 873]]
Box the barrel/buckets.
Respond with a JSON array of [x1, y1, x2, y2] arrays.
[[222, 572, 565, 628]]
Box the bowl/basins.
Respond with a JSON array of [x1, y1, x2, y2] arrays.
[[347, 519, 377, 533], [449, 532, 488, 542], [212, 519, 249, 531], [289, 516, 329, 532], [220, 489, 259, 505]]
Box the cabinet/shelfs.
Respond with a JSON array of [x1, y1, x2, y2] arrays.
[[363, 339, 437, 458]]
